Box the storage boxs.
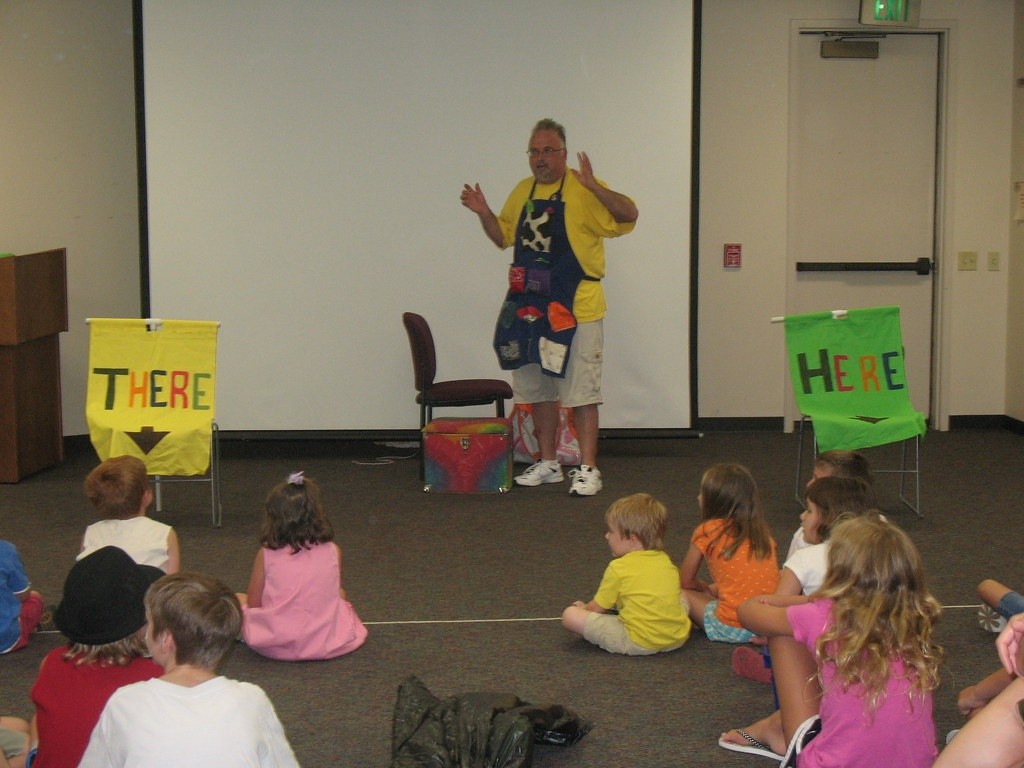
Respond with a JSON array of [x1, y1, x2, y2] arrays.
[[419, 417, 513, 495], [509, 403, 583, 467]]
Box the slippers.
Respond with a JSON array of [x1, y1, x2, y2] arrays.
[[718, 728, 786, 762]]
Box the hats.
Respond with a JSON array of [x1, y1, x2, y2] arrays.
[[52, 546, 166, 644]]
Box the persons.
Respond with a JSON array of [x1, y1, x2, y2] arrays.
[[562, 493, 692, 655], [235, 471, 369, 662], [27, 546, 168, 768], [460, 119, 639, 497], [0, 715, 29, 768], [0, 539, 44, 654], [718, 449, 945, 768], [75, 455, 179, 575], [932, 579, 1024, 768], [77, 572, 301, 768], [678, 465, 780, 643]]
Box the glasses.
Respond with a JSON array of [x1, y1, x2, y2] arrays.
[[526, 147, 562, 157]]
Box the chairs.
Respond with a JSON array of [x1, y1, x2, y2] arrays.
[[81, 315, 225, 529], [402, 312, 514, 482], [769, 305, 928, 521]]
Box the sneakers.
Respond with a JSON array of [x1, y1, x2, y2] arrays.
[[513, 458, 566, 487], [567, 464, 603, 497]]
